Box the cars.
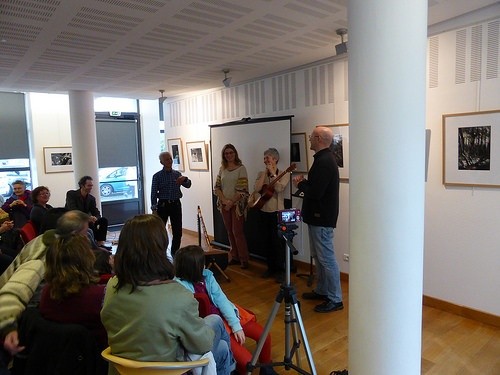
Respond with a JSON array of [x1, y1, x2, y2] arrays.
[[99, 166, 137, 198], [0, 166, 31, 197]]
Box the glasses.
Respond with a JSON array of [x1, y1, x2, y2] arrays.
[[309, 134, 318, 138]]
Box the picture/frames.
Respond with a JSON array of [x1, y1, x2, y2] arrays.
[[316, 123, 350, 182], [291, 132, 310, 173], [167, 138, 185, 173], [186, 141, 209, 172], [43, 147, 74, 173], [442, 109, 500, 188]]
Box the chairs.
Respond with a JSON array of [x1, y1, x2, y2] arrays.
[[101, 345, 210, 375]]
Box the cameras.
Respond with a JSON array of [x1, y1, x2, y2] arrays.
[[278, 208, 301, 224]]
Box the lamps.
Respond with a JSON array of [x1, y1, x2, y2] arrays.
[[222, 68, 231, 86], [335, 28, 349, 55]]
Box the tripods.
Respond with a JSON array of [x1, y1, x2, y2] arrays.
[[245, 223, 317, 375]]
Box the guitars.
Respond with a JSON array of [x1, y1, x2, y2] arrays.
[[247, 163, 297, 208]]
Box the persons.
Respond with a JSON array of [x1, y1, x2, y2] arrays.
[[215, 142, 251, 269], [174, 245, 279, 375], [254, 148, 298, 282], [64, 176, 112, 250], [292, 127, 344, 312], [60, 153, 72, 165], [101, 214, 235, 375], [30, 186, 58, 237], [1, 180, 34, 214], [0, 204, 114, 375], [151, 152, 192, 260]]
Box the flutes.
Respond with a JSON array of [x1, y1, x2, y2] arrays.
[[198, 206, 210, 244]]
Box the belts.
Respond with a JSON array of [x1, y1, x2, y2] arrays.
[[168, 199, 174, 203]]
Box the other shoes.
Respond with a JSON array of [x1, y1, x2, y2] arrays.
[[313, 301, 344, 312], [230, 259, 241, 265], [98, 244, 112, 250], [260, 365, 279, 375], [240, 263, 248, 268], [301, 289, 327, 300]]
